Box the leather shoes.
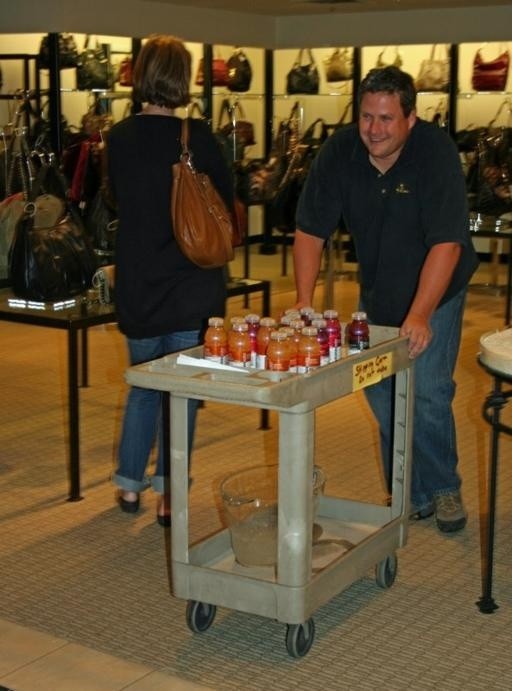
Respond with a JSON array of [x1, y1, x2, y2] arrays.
[[157, 514, 171, 527], [118, 492, 139, 513]]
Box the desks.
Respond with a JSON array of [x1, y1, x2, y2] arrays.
[[2, 274, 271, 502], [472, 330, 511, 617]]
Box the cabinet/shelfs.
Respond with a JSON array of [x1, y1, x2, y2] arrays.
[[269, 40, 510, 264], [0, 31, 268, 255]]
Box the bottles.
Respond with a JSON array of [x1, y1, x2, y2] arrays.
[[204, 306, 370, 374]]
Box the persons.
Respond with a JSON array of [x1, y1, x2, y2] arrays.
[[103, 31, 235, 528], [289, 62, 474, 535]]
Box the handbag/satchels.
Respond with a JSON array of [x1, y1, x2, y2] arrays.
[[286, 49, 319, 94], [195, 45, 253, 92], [414, 43, 452, 92], [0, 191, 65, 282], [171, 117, 235, 269], [36, 33, 134, 89], [240, 100, 322, 234], [376, 52, 403, 70], [326, 47, 354, 82], [217, 119, 255, 146], [92, 264, 116, 305], [10, 204, 95, 305], [454, 100, 512, 215], [80, 111, 115, 139], [472, 42, 509, 91]]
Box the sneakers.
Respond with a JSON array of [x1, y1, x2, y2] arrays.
[[433, 487, 466, 533], [388, 502, 434, 521]]
